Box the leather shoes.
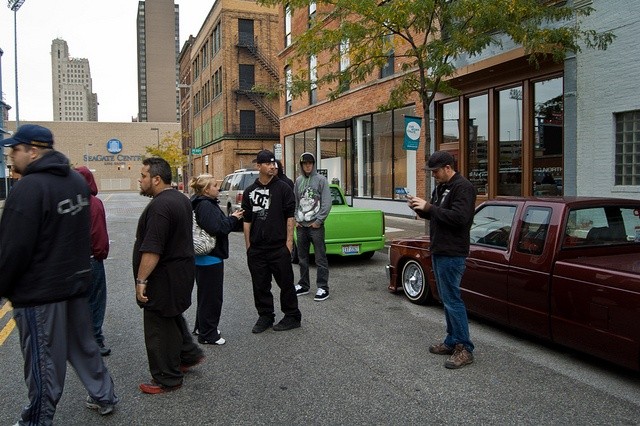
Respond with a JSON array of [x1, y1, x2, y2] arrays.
[[273, 315, 301, 331], [252, 316, 274, 334]]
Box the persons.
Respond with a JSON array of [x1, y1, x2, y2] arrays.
[[241, 149, 302, 333], [293, 151, 333, 301], [72, 165, 111, 355], [405, 151, 477, 369], [274, 158, 292, 192], [0, 124, 118, 426], [189, 173, 245, 345], [133, 157, 204, 393]]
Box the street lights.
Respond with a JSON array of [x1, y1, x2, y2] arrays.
[[150, 126, 161, 158], [178, 83, 193, 180], [6, 0, 25, 133], [86, 143, 92, 169]]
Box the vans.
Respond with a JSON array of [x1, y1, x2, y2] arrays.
[[215, 168, 263, 223]]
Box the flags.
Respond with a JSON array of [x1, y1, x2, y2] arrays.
[[403, 117, 422, 149]]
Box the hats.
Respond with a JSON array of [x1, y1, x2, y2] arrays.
[[252, 150, 275, 164], [301, 155, 315, 163], [421, 151, 453, 171], [1, 124, 53, 149]]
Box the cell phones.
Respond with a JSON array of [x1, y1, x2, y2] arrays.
[[404, 188, 414, 197]]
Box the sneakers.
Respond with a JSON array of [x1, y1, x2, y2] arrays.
[[295, 283, 310, 297], [193, 329, 221, 335], [85, 394, 115, 416], [98, 346, 111, 356], [198, 336, 226, 346], [445, 344, 474, 369], [313, 287, 330, 302], [429, 343, 455, 356], [139, 379, 184, 394]]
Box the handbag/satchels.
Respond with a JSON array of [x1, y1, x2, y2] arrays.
[[192, 209, 216, 255]]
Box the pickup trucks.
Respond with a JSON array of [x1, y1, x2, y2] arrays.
[[384, 195, 638, 349], [291, 182, 385, 266]]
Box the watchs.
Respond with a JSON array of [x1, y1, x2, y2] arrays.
[[135, 278, 148, 285]]
